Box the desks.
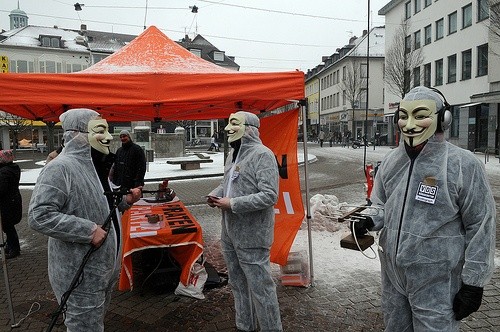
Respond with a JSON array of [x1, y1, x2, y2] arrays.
[[118, 196, 208, 299]]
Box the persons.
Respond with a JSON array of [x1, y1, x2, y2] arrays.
[[350, 85, 498, 332], [45, 129, 146, 215], [299, 129, 351, 147], [0, 150, 23, 259], [206, 111, 284, 332], [211, 135, 220, 151], [27, 109, 141, 332]]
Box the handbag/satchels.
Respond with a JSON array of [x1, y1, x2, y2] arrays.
[[175, 262, 228, 300]]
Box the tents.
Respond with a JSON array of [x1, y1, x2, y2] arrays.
[[0, 25, 314, 325]]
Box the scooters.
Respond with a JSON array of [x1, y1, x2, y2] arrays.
[[350, 136, 370, 149]]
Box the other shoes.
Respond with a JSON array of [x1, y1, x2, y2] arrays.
[[6, 250, 20, 259], [0, 249, 8, 254]]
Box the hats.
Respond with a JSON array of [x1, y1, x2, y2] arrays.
[[0, 149, 13, 163], [119, 130, 130, 138]]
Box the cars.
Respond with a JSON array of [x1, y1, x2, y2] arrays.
[[371, 133, 388, 146]]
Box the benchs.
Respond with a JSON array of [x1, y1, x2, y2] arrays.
[[167, 159, 213, 170]]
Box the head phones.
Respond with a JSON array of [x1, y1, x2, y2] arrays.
[[392, 87, 453, 132]]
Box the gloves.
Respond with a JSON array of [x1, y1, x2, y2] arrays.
[[349, 215, 373, 238], [452, 284, 482, 321]]
